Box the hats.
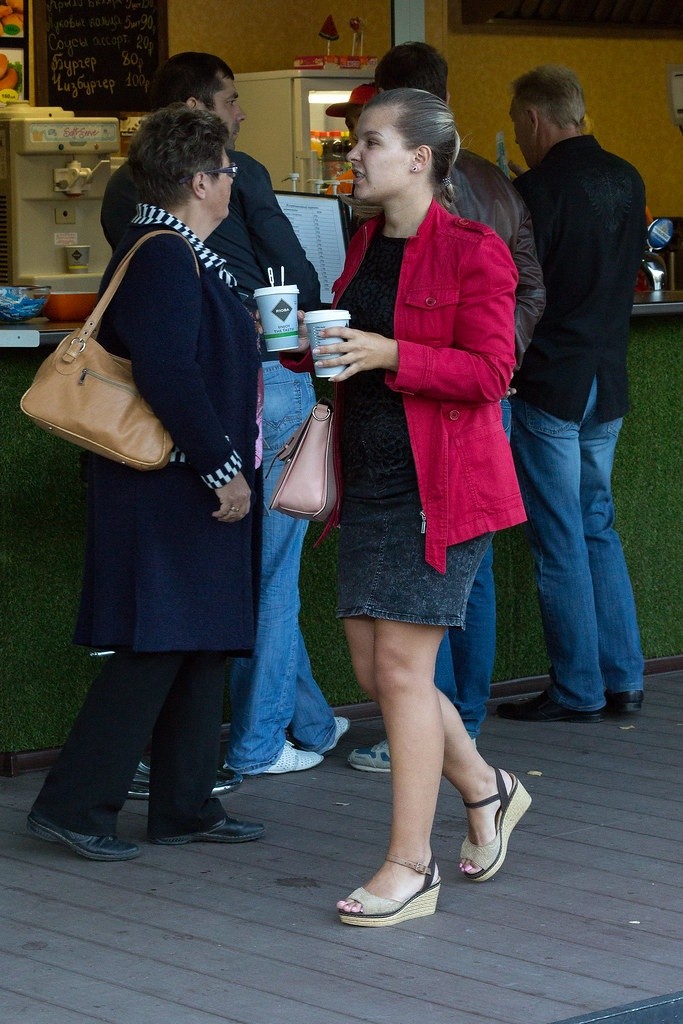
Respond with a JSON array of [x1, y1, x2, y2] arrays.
[[325, 84, 378, 117]]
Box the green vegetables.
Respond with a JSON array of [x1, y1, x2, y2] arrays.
[[8, 61, 23, 94]]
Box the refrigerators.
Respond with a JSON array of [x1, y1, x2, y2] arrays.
[[230, 70, 376, 192]]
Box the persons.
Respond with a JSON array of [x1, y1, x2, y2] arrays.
[[326, 83, 377, 195], [349, 35, 545, 774], [255, 88, 534, 927], [30, 109, 264, 860], [101, 53, 351, 774], [497, 65, 646, 720]]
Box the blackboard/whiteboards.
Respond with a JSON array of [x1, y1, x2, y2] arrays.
[[44, 0, 169, 116]]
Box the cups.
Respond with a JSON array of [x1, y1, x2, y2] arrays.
[[253, 284, 301, 352], [634, 250, 668, 292], [303, 308, 352, 378], [65, 245, 91, 274]]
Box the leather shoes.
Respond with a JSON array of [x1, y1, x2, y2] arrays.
[[149, 816, 266, 846], [497, 689, 604, 723], [605, 688, 645, 713], [26, 812, 140, 861]]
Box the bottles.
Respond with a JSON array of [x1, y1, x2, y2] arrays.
[[310, 130, 357, 194]]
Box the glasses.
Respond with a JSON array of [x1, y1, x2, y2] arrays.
[[178, 162, 237, 184]]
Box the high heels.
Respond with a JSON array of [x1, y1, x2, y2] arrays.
[[339, 853, 441, 928], [460, 765, 532, 882]]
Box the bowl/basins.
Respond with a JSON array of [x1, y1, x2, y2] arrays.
[[0, 286, 51, 325], [33, 291, 99, 322]]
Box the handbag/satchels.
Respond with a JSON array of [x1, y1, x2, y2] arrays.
[[20, 229, 199, 472], [264, 396, 334, 523]]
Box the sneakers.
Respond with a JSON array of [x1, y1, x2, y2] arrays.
[[127, 751, 244, 799], [328, 717, 350, 749], [347, 738, 391, 773], [260, 739, 324, 774]]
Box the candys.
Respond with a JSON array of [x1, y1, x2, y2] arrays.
[[349, 15, 368, 33]]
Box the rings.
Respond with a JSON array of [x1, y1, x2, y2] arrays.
[[230, 507, 239, 512]]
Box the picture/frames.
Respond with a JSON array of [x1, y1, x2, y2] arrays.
[[448, 0, 683, 41]]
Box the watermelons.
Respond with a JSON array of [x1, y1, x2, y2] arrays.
[[318, 14, 340, 41]]
